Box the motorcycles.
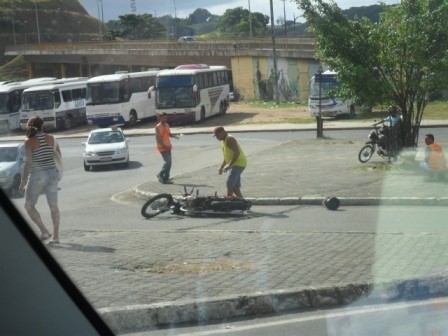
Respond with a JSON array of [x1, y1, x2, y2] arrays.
[[358, 122, 418, 164]]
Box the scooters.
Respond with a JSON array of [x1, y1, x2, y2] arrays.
[[140, 187, 253, 217]]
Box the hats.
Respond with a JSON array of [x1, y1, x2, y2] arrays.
[[212, 127, 224, 137]]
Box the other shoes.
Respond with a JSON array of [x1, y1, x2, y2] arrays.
[[157, 174, 164, 182], [39, 233, 51, 241], [46, 240, 59, 245], [163, 181, 173, 184]]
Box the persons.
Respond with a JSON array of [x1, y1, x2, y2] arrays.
[[19, 116, 63, 244], [154, 112, 179, 184], [419, 134, 445, 182], [212, 127, 246, 197], [373, 106, 402, 129]]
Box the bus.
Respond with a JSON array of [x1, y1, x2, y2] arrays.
[[87, 69, 158, 130], [0, 77, 88, 135], [157, 65, 233, 127], [307, 69, 374, 120], [20, 81, 88, 132]]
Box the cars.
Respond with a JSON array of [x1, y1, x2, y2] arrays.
[[82, 127, 131, 171], [179, 36, 196, 43], [0, 142, 25, 195]]
